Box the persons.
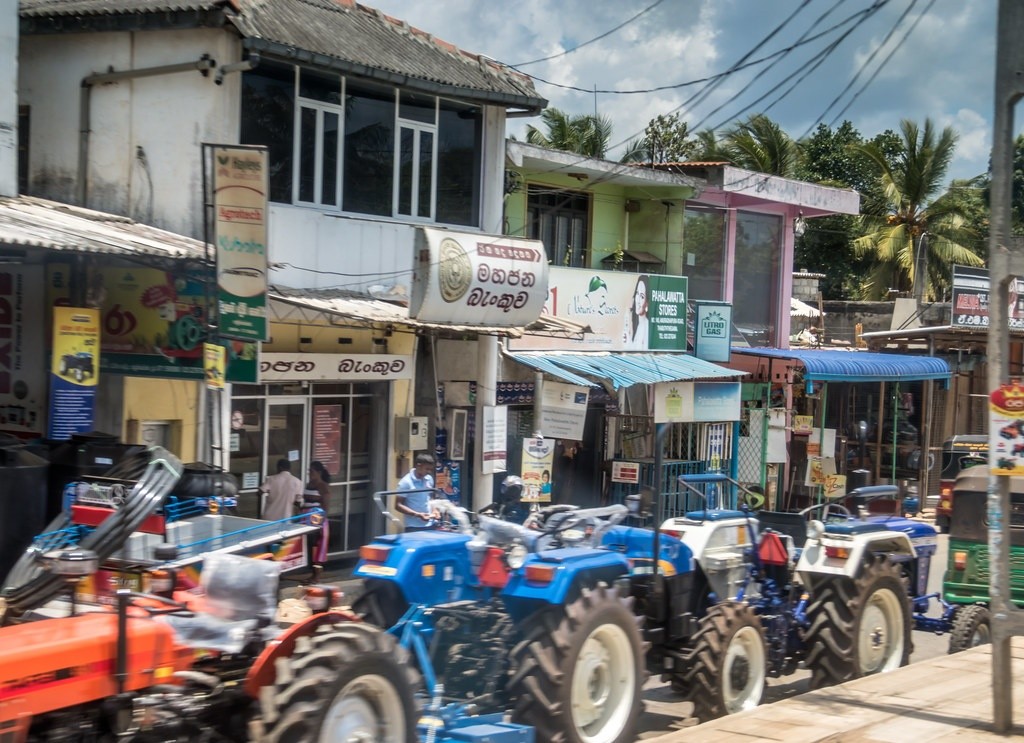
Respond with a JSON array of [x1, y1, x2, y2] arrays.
[[497, 474, 529, 526], [256, 458, 305, 525], [624, 275, 649, 350], [295, 461, 332, 581], [395, 454, 435, 532], [886, 393, 918, 469], [541, 470, 551, 493], [742, 487, 765, 510]]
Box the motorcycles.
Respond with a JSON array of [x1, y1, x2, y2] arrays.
[[935, 434, 1024, 606]]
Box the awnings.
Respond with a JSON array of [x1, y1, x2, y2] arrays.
[[0, 193, 217, 265], [272, 286, 594, 344], [733, 346, 953, 395], [509, 351, 751, 391]]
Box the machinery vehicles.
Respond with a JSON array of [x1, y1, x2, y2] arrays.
[[0, 418, 991, 743]]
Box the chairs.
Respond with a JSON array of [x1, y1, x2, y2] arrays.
[[478, 517, 551, 551], [166, 554, 288, 653], [754, 511, 807, 546]]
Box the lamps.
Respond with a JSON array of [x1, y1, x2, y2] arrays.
[[794, 209, 809, 237]]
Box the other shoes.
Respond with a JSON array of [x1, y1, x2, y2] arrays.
[[300, 577, 320, 584]]
